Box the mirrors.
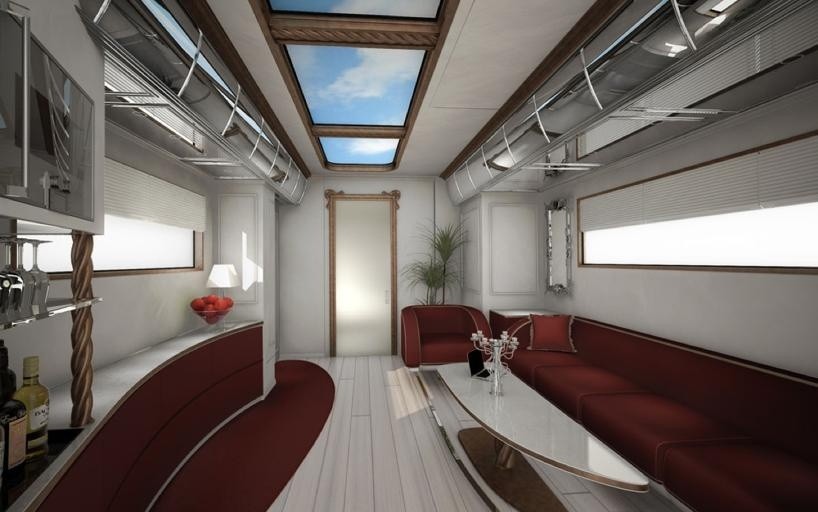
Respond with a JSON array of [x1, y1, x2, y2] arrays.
[[545, 200, 573, 297]]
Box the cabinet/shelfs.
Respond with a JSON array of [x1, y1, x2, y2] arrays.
[[1, 216, 94, 510]]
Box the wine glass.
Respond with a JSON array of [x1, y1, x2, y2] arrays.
[[0, 235, 51, 326], [193, 309, 231, 333]]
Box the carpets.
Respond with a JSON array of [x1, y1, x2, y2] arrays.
[[148, 360, 336, 511]]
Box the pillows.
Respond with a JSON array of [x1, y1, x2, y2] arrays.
[[526, 315, 577, 352]]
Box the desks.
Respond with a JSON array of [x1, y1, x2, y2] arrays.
[[436, 360, 651, 512]]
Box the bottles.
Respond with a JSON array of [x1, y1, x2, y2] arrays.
[[0, 338, 50, 476]]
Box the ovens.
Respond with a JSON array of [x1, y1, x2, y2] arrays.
[[0, 8, 97, 224]]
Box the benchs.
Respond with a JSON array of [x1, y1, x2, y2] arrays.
[[502, 314, 817, 507]]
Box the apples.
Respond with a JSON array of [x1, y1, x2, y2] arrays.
[[191, 294, 233, 324]]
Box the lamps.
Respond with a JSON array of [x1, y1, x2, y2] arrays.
[[205, 264, 240, 328]]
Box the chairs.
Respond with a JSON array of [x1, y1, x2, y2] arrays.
[[401, 304, 495, 372]]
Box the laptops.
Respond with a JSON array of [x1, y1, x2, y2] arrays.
[[466, 348, 506, 382]]
